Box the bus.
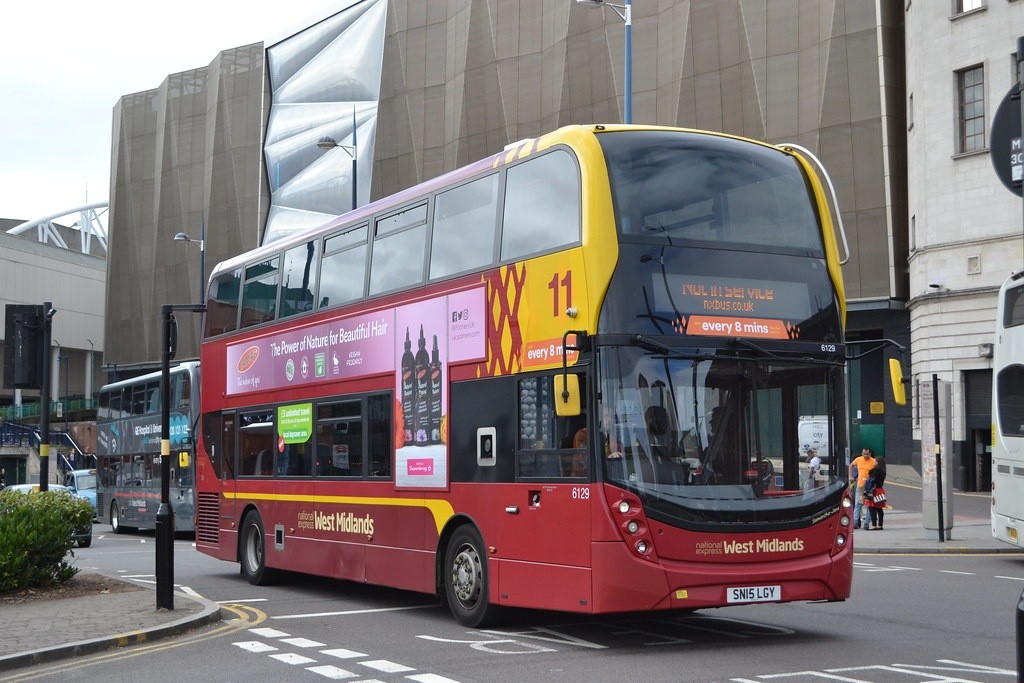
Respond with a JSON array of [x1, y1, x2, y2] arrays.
[[96, 361, 201, 533], [196, 123, 912, 631], [991, 268, 1024, 549]]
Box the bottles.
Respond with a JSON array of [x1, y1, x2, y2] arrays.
[[414, 324, 430, 446], [402, 327, 414, 446], [430, 335, 442, 445]]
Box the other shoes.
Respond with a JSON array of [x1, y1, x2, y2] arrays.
[[863, 524, 869, 529], [876, 527, 883, 530], [866, 527, 877, 530], [854, 524, 861, 528]]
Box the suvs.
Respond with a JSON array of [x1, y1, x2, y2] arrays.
[[64, 470, 97, 519]]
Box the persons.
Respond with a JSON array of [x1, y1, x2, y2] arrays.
[[807, 449, 820, 479], [849, 448, 887, 530], [572, 407, 622, 477]]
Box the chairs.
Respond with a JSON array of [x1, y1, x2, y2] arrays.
[[238, 443, 333, 475]]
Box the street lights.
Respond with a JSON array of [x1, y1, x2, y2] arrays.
[[316, 104, 357, 210], [174, 209, 205, 322], [576, 0, 632, 122]]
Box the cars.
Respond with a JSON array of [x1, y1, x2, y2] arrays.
[[0, 484, 93, 548]]
